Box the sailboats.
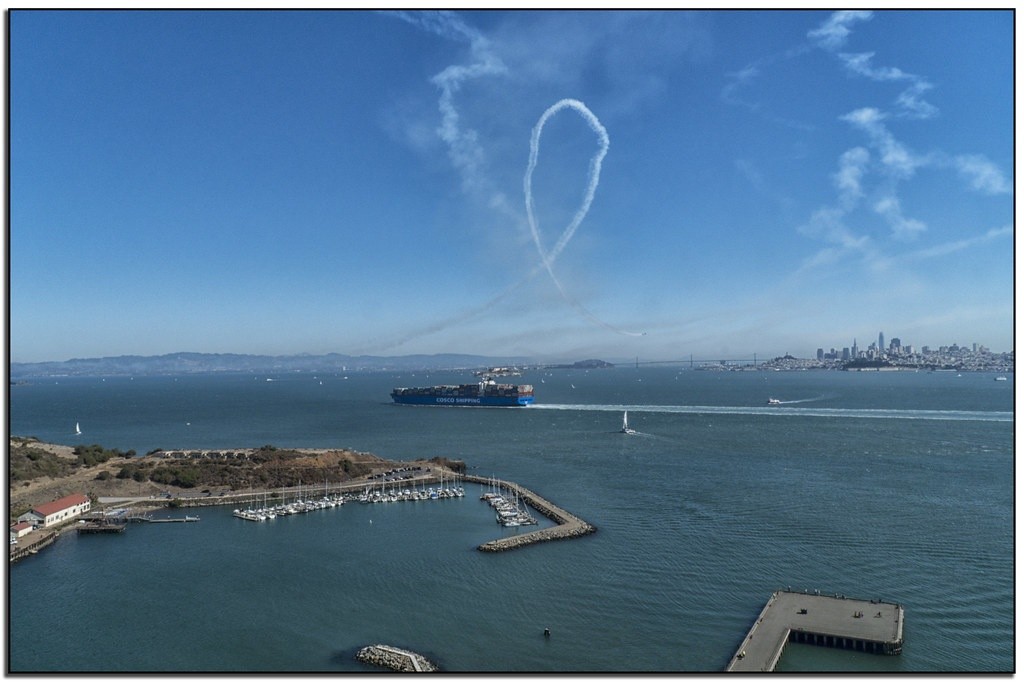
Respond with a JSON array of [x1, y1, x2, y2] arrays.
[[75, 422, 81, 435], [232, 466, 466, 518], [994, 376, 1006, 381], [478, 470, 539, 528], [767, 398, 780, 404], [621, 410, 636, 435]]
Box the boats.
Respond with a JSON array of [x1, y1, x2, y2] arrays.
[[390, 382, 535, 406], [73, 505, 128, 536]]
